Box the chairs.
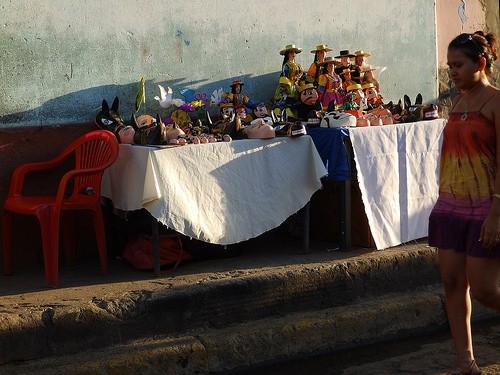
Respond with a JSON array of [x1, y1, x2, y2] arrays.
[[2, 130, 120, 288]]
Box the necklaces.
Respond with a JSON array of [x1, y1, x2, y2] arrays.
[[461, 82, 491, 121]]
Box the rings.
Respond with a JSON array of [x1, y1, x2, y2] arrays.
[[496, 238, 500, 241]]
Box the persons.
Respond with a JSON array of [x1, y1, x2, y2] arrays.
[[429, 30, 500, 375]]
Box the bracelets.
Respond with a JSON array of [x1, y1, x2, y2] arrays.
[[493, 194, 500, 199]]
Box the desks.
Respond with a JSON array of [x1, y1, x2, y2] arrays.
[[101, 135, 328, 255], [306, 118, 448, 250]]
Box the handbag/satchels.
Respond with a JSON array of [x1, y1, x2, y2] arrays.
[[122, 233, 192, 274]]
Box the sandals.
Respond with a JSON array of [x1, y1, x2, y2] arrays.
[[441, 359, 481, 375]]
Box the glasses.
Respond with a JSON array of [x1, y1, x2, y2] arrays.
[[453, 33, 485, 56]]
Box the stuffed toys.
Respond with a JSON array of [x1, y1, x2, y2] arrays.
[[96, 44, 441, 145]]
